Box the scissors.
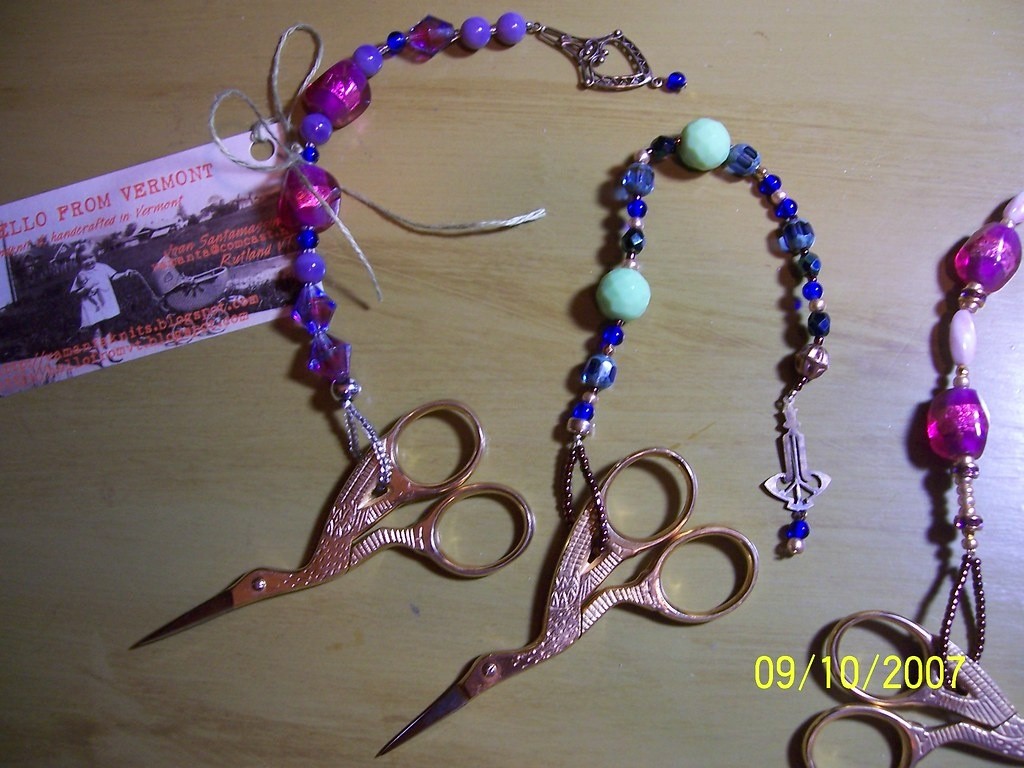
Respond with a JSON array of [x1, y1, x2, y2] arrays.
[[801, 609, 1024, 768], [372, 443, 756, 762], [126, 397, 537, 650]]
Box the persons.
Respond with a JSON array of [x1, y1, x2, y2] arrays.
[[68, 243, 136, 366]]
